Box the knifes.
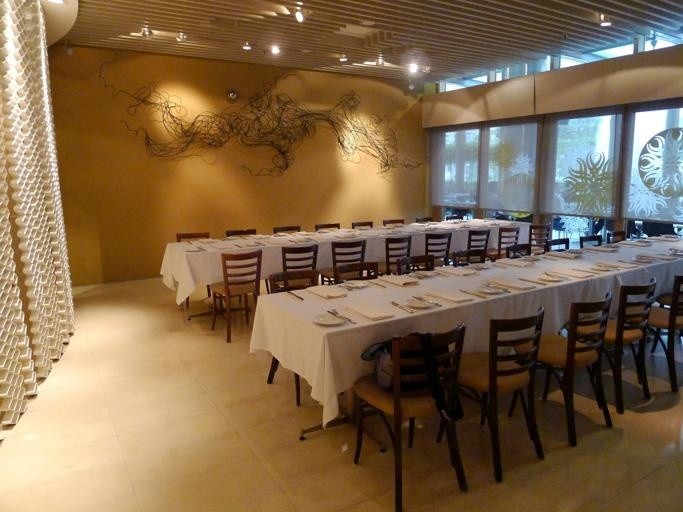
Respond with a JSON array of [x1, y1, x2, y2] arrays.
[[390, 302, 413, 314], [327, 309, 357, 326]]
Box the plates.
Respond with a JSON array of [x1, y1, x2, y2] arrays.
[[184, 216, 501, 252], [414, 271, 437, 278], [342, 282, 370, 289], [405, 299, 436, 309], [313, 314, 347, 326], [435, 233, 682, 326]]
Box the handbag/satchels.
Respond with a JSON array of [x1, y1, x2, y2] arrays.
[[362, 321, 467, 421]]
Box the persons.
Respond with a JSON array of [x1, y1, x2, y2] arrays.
[[485, 182, 504, 209], [553, 183, 565, 212]]
